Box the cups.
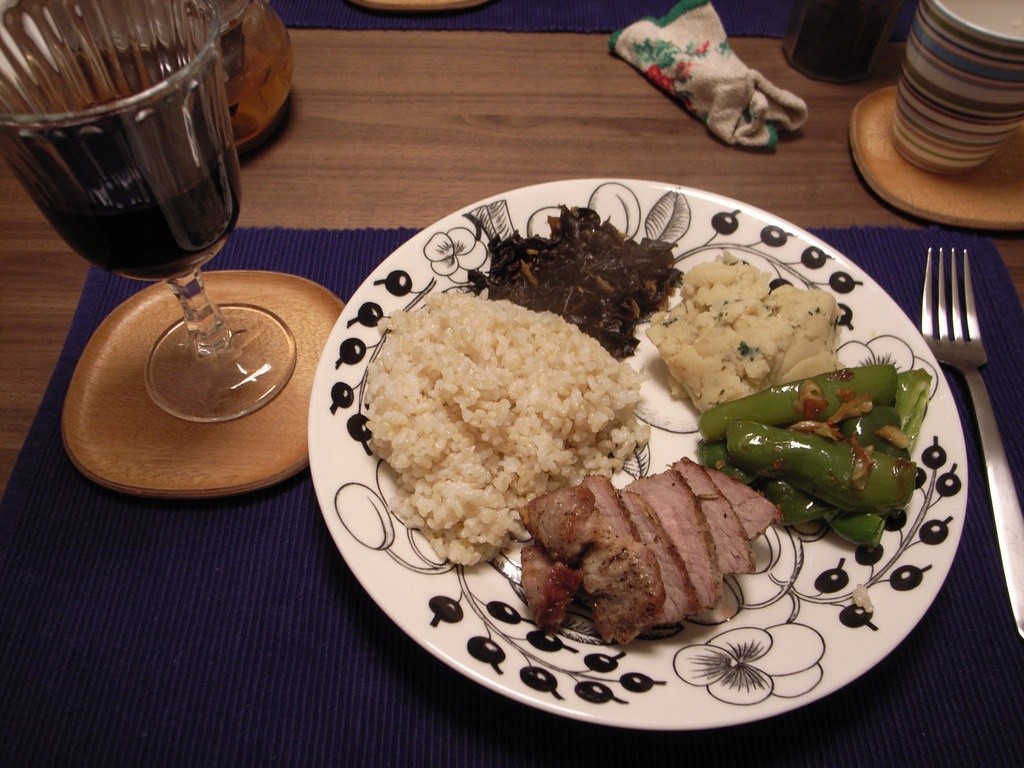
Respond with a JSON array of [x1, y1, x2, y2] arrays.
[[893, 0, 1024, 176], [783, 0, 910, 82]]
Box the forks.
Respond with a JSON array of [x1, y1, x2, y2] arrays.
[[922, 247, 1024, 639]]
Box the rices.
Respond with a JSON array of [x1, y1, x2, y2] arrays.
[[363, 288, 651, 568]]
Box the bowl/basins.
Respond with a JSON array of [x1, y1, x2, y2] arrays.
[[209, 0, 294, 156]]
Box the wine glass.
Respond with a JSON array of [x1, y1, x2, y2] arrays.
[[0, 0, 296, 423]]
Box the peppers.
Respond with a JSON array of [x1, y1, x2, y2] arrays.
[[697, 362, 934, 549]]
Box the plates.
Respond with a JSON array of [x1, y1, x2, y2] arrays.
[[306, 178, 968, 728]]
